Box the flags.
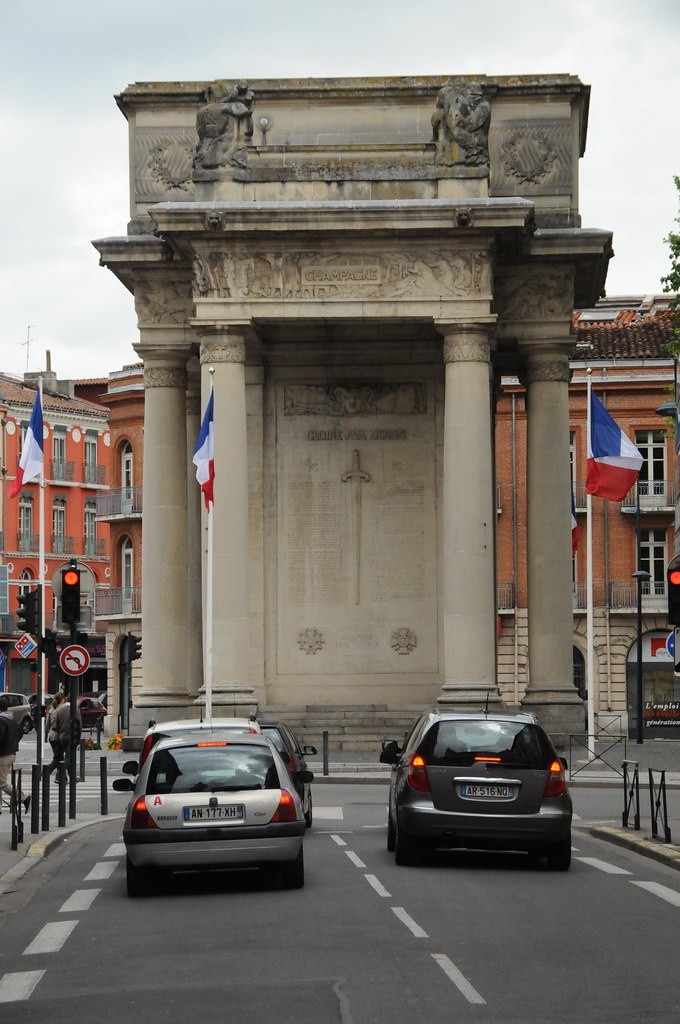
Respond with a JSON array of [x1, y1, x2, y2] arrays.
[[193, 386, 215, 513], [9, 387, 43, 499], [585, 384, 644, 502]]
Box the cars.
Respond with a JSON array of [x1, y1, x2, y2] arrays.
[[112, 713, 317, 891], [0, 691, 108, 734]]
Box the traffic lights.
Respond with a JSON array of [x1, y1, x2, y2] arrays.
[[666, 553, 680, 622], [61, 569, 80, 623], [14, 591, 37, 635]]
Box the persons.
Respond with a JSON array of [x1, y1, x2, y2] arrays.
[[45, 692, 81, 785], [0, 699, 31, 815]]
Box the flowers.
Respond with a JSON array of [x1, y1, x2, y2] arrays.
[[105, 733, 125, 750]]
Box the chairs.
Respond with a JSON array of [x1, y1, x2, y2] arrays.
[[497, 727, 526, 748], [433, 725, 467, 757]]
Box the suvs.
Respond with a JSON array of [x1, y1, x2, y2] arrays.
[[379, 708, 574, 869]]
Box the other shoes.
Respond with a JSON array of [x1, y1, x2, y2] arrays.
[[54, 778, 67, 785], [70, 778, 82, 784], [23, 795, 31, 814]]
